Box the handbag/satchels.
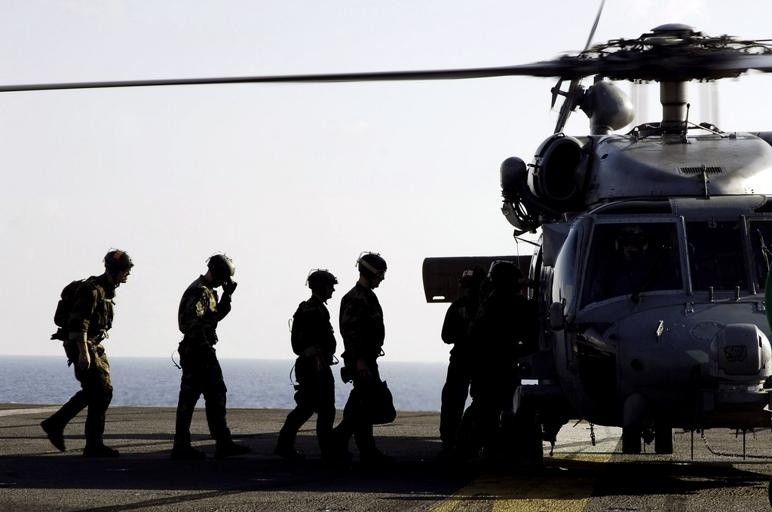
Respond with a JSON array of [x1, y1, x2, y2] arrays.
[[372, 379, 396, 424]]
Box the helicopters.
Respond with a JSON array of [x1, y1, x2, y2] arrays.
[[0, 2, 771, 454]]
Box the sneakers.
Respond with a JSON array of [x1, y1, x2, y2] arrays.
[[219, 443, 250, 457], [275, 448, 386, 465], [40, 418, 66, 453], [171, 447, 206, 462], [82, 444, 119, 458]]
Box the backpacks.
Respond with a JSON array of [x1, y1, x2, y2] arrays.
[[53, 272, 117, 329]]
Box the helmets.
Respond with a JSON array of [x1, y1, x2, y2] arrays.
[[358, 253, 387, 275], [307, 271, 339, 289], [104, 250, 134, 269], [207, 254, 236, 277], [487, 260, 524, 283], [456, 263, 487, 288]]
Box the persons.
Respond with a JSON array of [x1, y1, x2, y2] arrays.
[[432, 262, 492, 467], [449, 261, 542, 475], [38, 250, 132, 456], [276, 270, 337, 461], [326, 254, 399, 468], [169, 255, 255, 461]]
